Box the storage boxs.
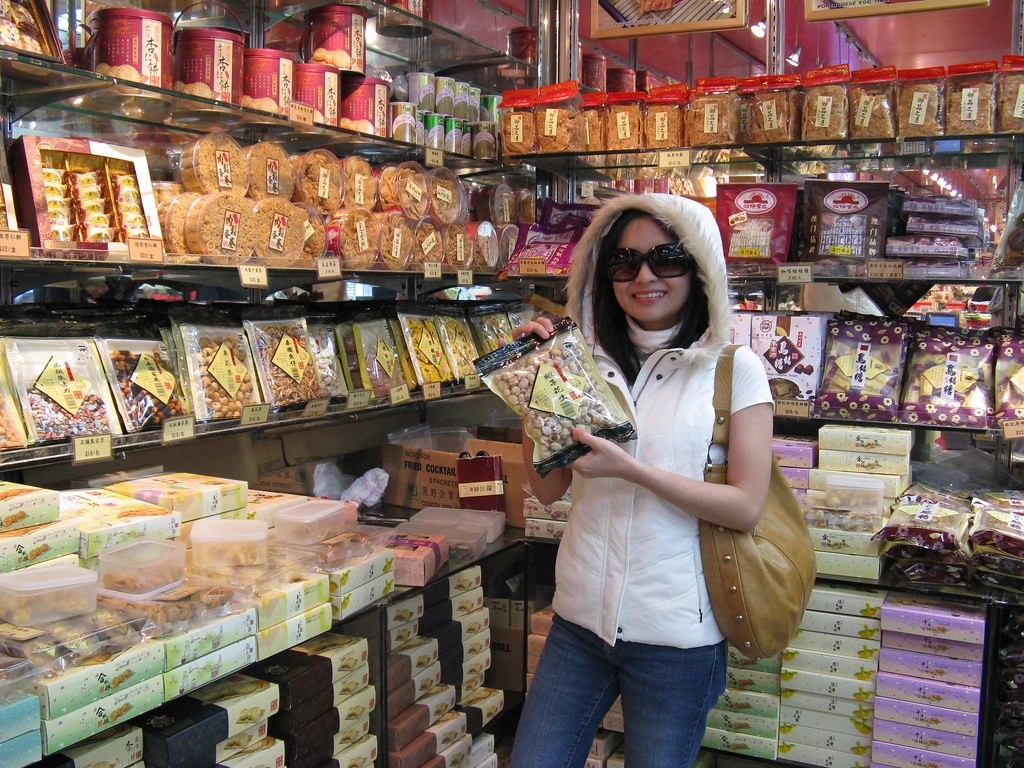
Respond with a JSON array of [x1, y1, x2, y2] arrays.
[[0, 421, 572, 768], [527, 424, 986, 767]]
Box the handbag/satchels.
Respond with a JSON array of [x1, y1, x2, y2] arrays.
[[698, 344, 816, 659]]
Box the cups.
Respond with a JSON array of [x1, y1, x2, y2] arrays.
[[389, 102, 416, 144], [470, 121, 501, 159], [444, 118, 462, 153], [415, 111, 433, 145], [469, 87, 481, 122], [435, 77, 455, 116], [425, 114, 445, 149], [481, 95, 514, 132], [152, 182, 185, 253], [455, 82, 470, 121], [407, 73, 435, 113], [462, 122, 471, 156]]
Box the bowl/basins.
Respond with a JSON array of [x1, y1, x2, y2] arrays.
[[180, 133, 537, 228], [158, 191, 521, 274]]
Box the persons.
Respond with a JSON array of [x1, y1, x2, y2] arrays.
[[499, 192, 776, 768]]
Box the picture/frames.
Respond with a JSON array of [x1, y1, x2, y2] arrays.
[[590, 0, 747, 38], [804, 0, 989, 21]]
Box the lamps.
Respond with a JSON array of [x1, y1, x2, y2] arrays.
[[786, 0, 802, 67], [750, 18, 767, 38], [815, 23, 825, 69]]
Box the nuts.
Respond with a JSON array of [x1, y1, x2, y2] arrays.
[[494, 346, 618, 459], [0, 326, 260, 448]]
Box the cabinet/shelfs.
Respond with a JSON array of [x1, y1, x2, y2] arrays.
[[543, 0, 1024, 768], [0, 0, 539, 768]]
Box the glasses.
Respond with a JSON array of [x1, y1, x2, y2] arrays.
[[601, 242, 693, 282]]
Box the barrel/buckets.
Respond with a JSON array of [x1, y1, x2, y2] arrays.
[[172, 1, 243, 126], [578, 41, 609, 93], [80, 8, 175, 122], [275, 63, 340, 139], [236, 48, 296, 134], [376, 0, 433, 38], [606, 67, 636, 92], [298, 5, 367, 76], [506, 26, 537, 65], [342, 76, 390, 145]]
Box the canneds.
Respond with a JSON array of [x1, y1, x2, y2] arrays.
[[573, 41, 666, 91], [81, 0, 539, 160]]
